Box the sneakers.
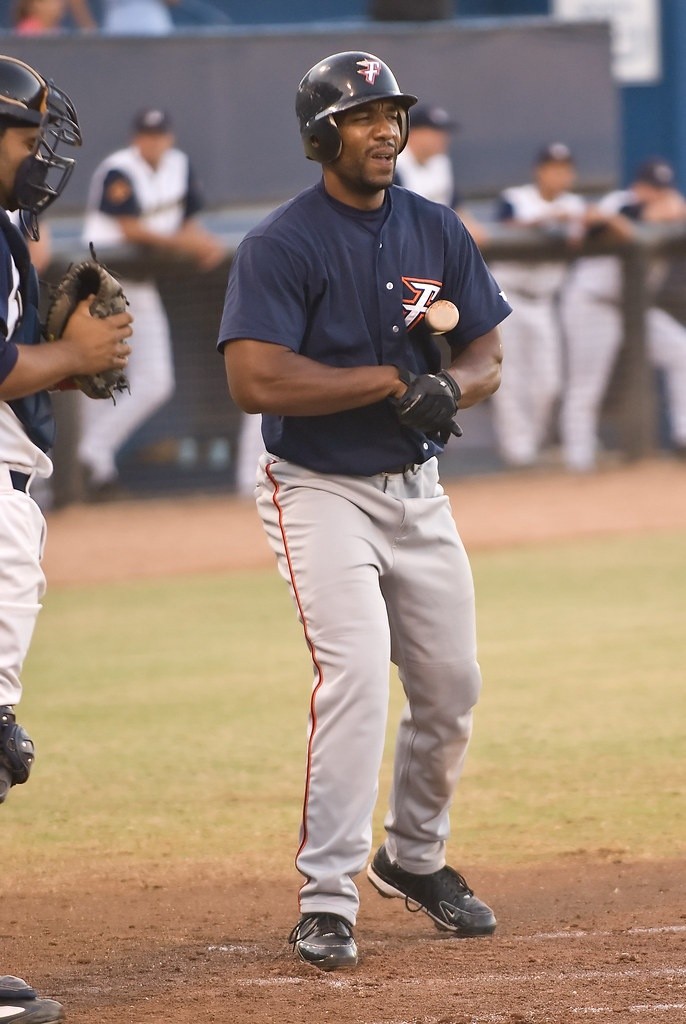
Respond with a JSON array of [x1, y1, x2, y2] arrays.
[[366, 842, 497, 938], [287, 913, 358, 972], [0, 976, 67, 1024]]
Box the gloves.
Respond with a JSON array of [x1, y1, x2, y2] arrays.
[[384, 360, 462, 444]]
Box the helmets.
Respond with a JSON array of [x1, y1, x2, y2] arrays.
[[295, 51, 419, 164], [0, 54, 66, 125]]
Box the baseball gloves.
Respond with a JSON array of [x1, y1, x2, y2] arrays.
[[39, 239, 134, 408]]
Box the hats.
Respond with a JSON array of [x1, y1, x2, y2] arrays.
[[534, 142, 570, 160], [407, 105, 454, 130], [133, 108, 173, 132], [638, 159, 674, 188]]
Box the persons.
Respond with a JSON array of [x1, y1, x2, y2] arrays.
[[102, 0, 181, 37], [390, 101, 489, 251], [66, 105, 235, 502], [15, 0, 99, 34], [557, 156, 686, 481], [0, 49, 132, 1024], [217, 50, 511, 969], [483, 141, 591, 469]]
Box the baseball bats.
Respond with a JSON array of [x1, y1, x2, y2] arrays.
[[424, 299, 460, 337]]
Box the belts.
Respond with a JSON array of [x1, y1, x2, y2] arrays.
[[9, 469, 30, 493]]
